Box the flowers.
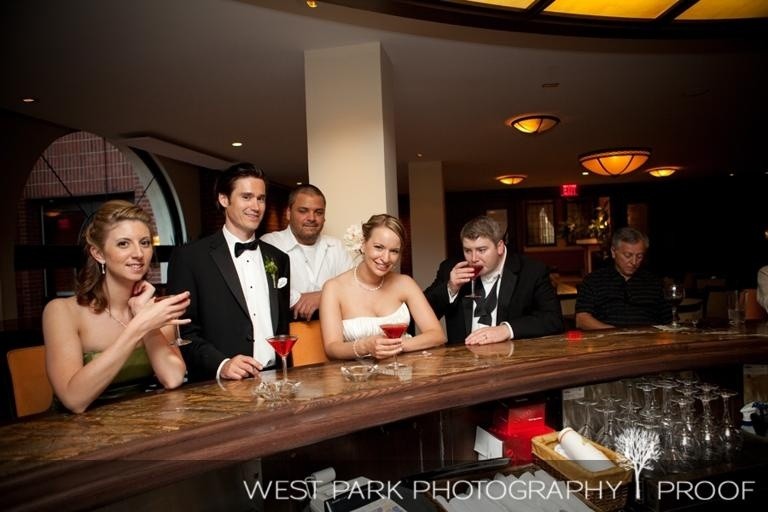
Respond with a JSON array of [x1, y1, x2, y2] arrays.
[[343, 224, 364, 254]]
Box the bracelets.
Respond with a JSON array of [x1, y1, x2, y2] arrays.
[[352, 339, 364, 357]]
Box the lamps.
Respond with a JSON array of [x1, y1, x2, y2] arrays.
[[578, 146, 651, 178], [644, 164, 682, 178], [497, 174, 527, 186], [512, 115, 561, 135]]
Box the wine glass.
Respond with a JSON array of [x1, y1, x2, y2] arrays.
[[156, 291, 194, 349], [663, 281, 686, 328], [462, 264, 484, 299], [264, 333, 299, 388], [379, 322, 410, 374], [573, 371, 745, 479]]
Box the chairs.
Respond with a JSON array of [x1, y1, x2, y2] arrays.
[[5, 346, 53, 417], [738, 288, 764, 320], [278, 313, 332, 368]]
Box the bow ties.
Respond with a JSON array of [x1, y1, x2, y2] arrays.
[[235, 241, 262, 258]]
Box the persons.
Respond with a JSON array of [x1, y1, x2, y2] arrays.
[[575, 226, 673, 329], [167, 163, 290, 383], [320, 213, 448, 360], [259, 184, 362, 321], [424, 217, 564, 345], [42, 200, 194, 415]]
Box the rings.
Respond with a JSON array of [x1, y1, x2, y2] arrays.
[[483, 335, 489, 340]]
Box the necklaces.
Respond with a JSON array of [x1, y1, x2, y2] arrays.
[[351, 264, 386, 290], [100, 305, 137, 328]]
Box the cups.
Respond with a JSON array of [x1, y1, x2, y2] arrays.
[[727, 289, 749, 327]]
